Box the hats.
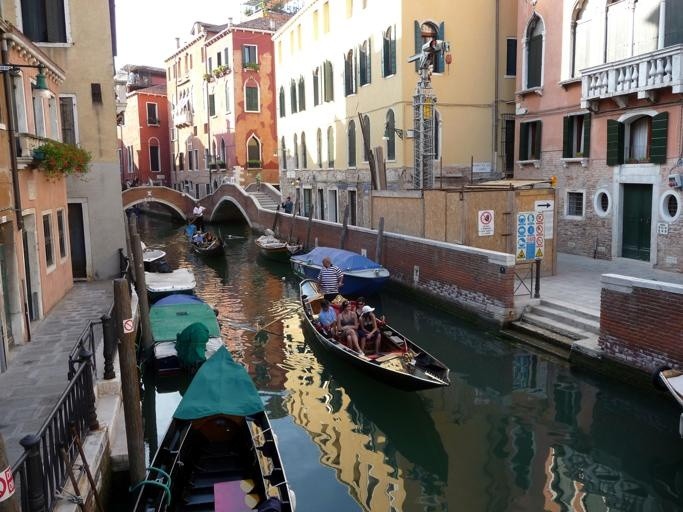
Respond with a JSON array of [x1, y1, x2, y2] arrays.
[[355, 296, 365, 303], [361, 305, 376, 314], [331, 294, 348, 304]]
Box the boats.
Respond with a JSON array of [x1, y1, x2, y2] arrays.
[[660, 369, 682, 406]]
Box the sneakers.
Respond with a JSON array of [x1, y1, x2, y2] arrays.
[[358, 350, 365, 356]]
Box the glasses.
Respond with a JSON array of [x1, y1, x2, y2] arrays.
[[343, 301, 351, 305], [356, 303, 363, 306]]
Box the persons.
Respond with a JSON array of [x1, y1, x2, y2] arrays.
[[280, 196, 293, 215], [319, 298, 337, 339], [336, 299, 365, 357], [356, 304, 381, 355], [192, 201, 207, 227], [192, 230, 212, 247], [352, 296, 367, 322], [183, 218, 197, 245], [316, 257, 343, 300]]
